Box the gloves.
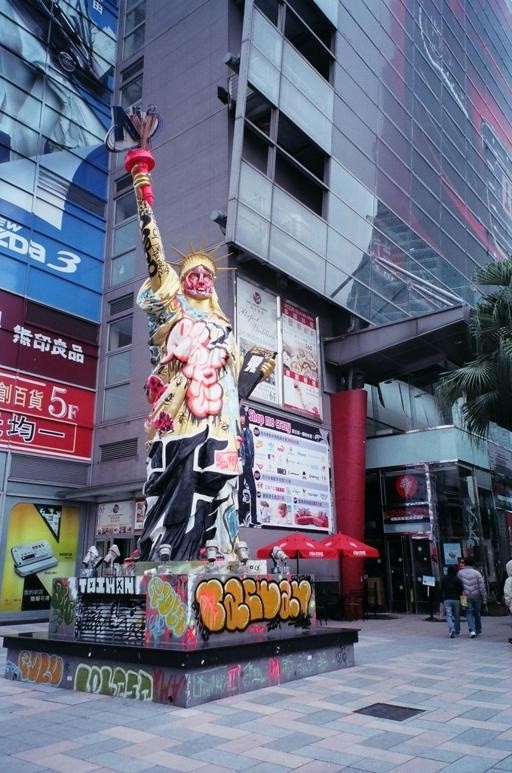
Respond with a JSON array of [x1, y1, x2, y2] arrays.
[[482, 600, 487, 605]]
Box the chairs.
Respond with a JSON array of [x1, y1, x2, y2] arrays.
[[344, 589, 377, 622]]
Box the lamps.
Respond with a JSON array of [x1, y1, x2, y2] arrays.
[[103, 544, 121, 572], [82, 545, 99, 569], [269, 545, 289, 574], [235, 541, 249, 568], [206, 539, 219, 568], [157, 543, 173, 565]]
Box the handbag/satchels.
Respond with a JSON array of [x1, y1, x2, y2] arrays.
[[460, 596, 468, 607]]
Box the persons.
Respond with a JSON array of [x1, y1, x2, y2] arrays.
[[117, 96, 280, 563], [235, 405, 262, 529], [504, 559, 512, 644], [457, 556, 489, 638], [438, 563, 466, 638]]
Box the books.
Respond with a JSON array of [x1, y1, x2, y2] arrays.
[[238, 342, 278, 400]]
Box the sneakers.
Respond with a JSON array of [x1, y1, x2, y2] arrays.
[[509, 638, 512, 644], [450, 629, 462, 638], [469, 631, 477, 638]]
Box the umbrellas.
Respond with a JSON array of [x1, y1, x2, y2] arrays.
[[318, 530, 380, 591], [257, 528, 340, 575]]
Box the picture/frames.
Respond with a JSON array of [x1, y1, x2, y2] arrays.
[[281, 296, 324, 424], [233, 268, 282, 411]]
